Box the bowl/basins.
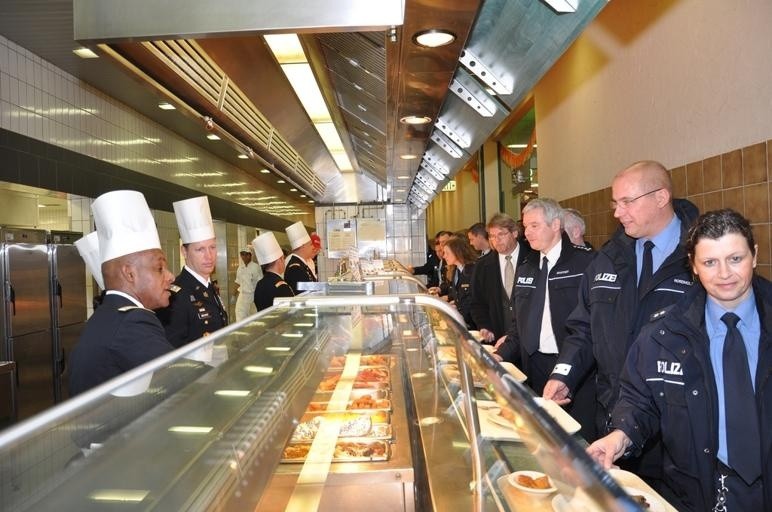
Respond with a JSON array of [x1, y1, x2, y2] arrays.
[[508, 470, 558, 498]]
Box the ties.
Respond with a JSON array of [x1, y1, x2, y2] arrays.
[[456, 269, 461, 287], [208, 282, 214, 296], [523, 256, 548, 356], [636, 240, 655, 301], [504, 256, 515, 299], [720, 311, 761, 486]]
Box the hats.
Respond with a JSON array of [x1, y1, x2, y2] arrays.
[[74, 231, 105, 291], [173, 195, 216, 244], [91, 190, 161, 263], [240, 248, 251, 253], [310, 235, 322, 249], [285, 220, 311, 250], [252, 231, 283, 266]]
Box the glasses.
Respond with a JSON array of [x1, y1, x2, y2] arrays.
[[610, 188, 662, 209], [488, 231, 509, 241]]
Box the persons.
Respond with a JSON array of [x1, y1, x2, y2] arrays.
[[67, 187, 229, 449], [231, 220, 322, 323], [406, 207, 595, 352], [541, 161, 702, 494], [585, 207, 772, 511], [480, 196, 598, 444]]
